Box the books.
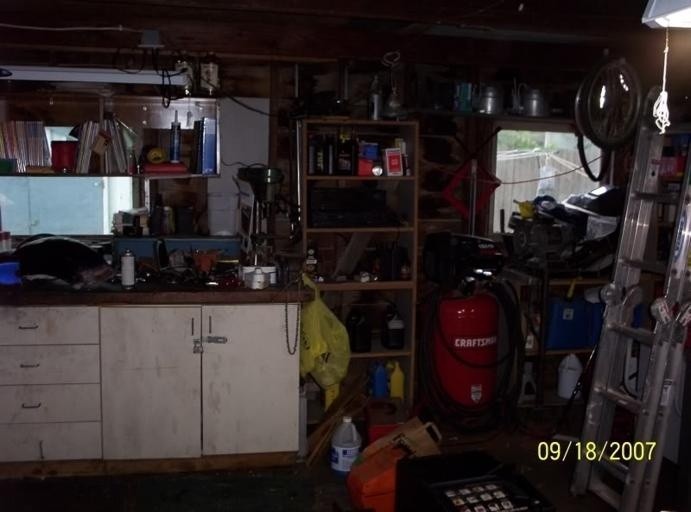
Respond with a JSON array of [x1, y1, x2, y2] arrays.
[[0, 114, 217, 177]]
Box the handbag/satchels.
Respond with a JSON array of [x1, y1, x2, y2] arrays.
[[347, 415, 443, 497]]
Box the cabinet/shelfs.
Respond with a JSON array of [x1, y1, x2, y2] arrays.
[[293, 119, 420, 425], [100, 304, 302, 461], [1, 89, 246, 272], [1, 299, 101, 460]]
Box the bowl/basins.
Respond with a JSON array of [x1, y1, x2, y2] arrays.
[[148, 148, 167, 163]]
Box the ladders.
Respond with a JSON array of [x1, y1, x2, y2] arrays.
[[568, 92, 688, 510]]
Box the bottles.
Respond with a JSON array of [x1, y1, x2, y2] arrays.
[[307, 137, 339, 177], [175, 49, 222, 97], [161, 205, 171, 237], [368, 91, 383, 121], [90, 242, 136, 291], [170, 110, 181, 163], [479, 87, 550, 120]]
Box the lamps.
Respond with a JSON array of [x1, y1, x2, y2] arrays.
[[113, 31, 190, 75], [640, 0, 691, 33]]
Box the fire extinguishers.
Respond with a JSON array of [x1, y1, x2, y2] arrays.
[[425, 273, 520, 429]]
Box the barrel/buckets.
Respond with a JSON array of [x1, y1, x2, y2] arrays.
[[557, 353, 582, 400], [331, 417, 361, 477], [375, 363, 386, 398], [386, 360, 404, 399]]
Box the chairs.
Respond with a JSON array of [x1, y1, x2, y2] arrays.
[[394, 449, 555, 512]]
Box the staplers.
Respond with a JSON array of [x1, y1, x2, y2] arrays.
[[0, 260, 22, 287]]
